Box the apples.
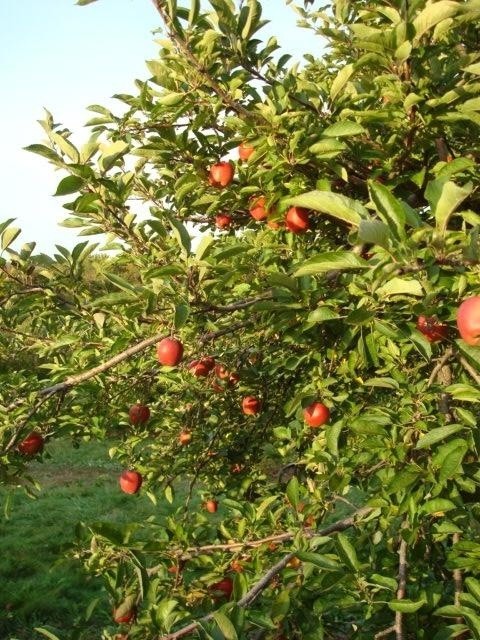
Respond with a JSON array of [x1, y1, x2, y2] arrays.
[[159, 339, 184, 365], [208, 137, 311, 234], [19, 432, 44, 456], [243, 396, 262, 415], [128, 405, 150, 424], [457, 296, 479, 347], [180, 433, 190, 444], [206, 501, 217, 513], [231, 466, 242, 474], [120, 470, 141, 495], [189, 356, 239, 391], [304, 402, 330, 428], [208, 577, 233, 604], [417, 316, 448, 342]]
